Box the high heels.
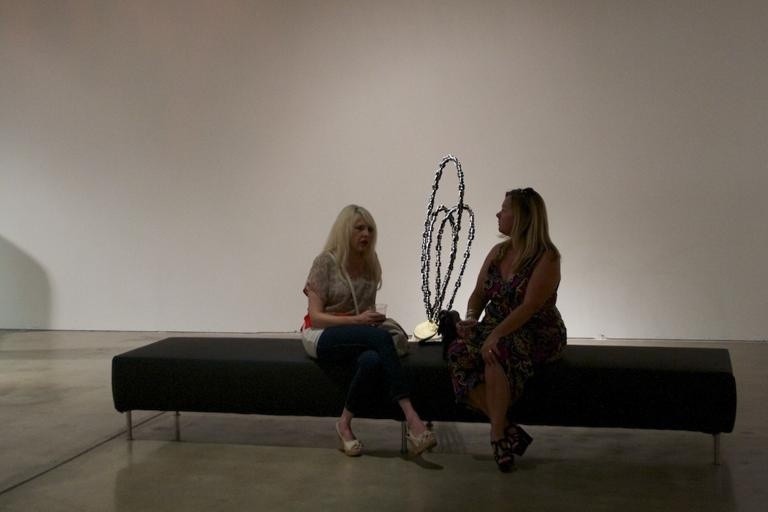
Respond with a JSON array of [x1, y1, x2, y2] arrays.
[[405, 424, 439, 461], [335, 421, 364, 456]]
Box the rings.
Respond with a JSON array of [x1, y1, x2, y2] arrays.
[[488, 350, 493, 354]]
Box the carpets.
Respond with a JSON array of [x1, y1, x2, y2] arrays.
[[0, 327, 166, 496]]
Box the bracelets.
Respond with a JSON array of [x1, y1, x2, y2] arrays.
[[466, 303, 483, 320]]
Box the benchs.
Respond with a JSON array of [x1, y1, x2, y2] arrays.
[[110, 335, 740, 468]]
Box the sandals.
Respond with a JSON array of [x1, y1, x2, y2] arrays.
[[490, 439, 516, 473], [509, 425, 532, 455]]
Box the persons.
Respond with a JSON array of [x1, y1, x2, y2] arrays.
[[445, 188, 567, 468], [301, 203, 436, 457]]
